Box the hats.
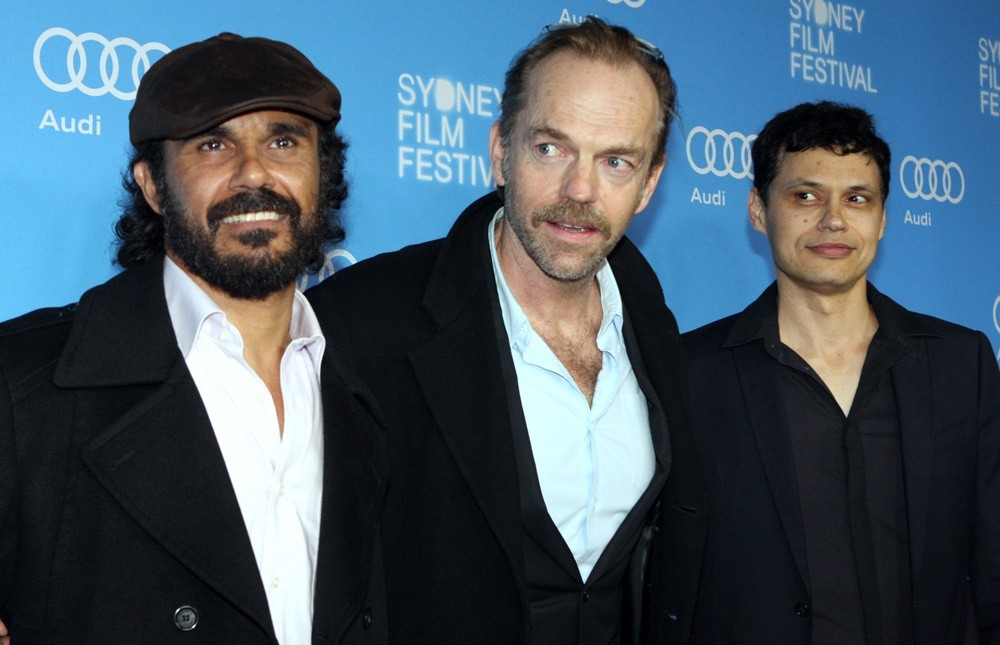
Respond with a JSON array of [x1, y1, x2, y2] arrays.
[[128, 30, 341, 155]]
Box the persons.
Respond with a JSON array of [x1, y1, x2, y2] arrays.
[[0, 29, 387, 645], [680, 100, 1000, 645], [304, 18, 736, 645]]
